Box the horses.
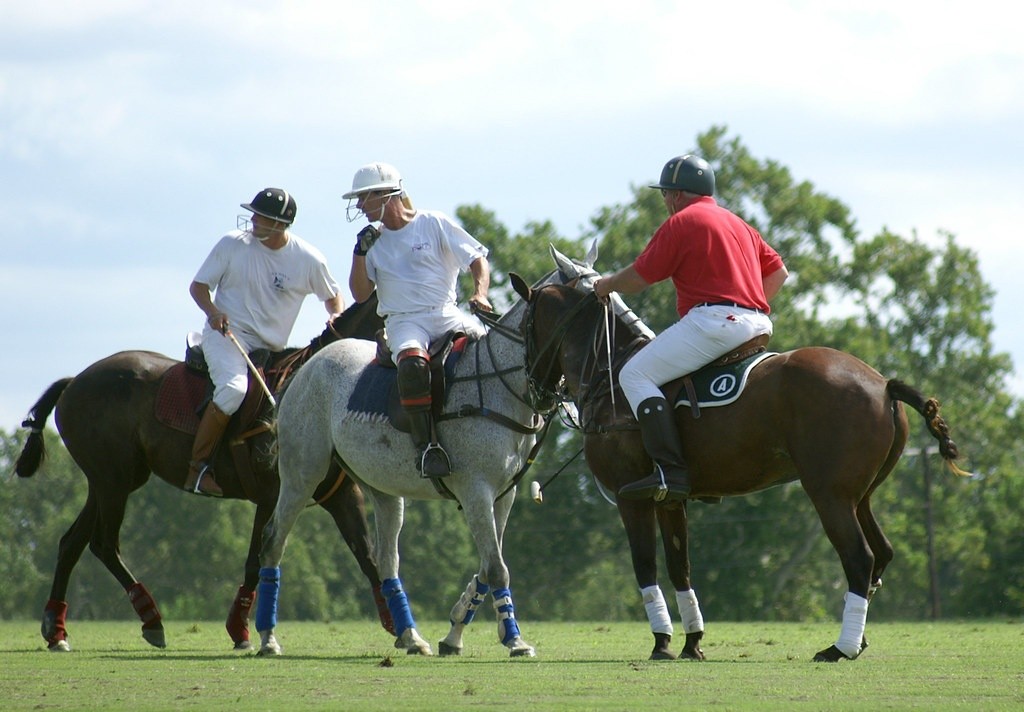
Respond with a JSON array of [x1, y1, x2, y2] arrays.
[[14, 237, 658, 659], [508, 272, 976, 664]]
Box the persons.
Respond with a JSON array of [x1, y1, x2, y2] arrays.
[[184, 188, 345, 496], [342, 161, 492, 478], [593, 154, 788, 502]]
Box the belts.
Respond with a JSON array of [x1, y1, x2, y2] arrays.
[[697, 299, 763, 315]]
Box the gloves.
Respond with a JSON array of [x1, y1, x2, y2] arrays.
[[354, 225, 382, 256]]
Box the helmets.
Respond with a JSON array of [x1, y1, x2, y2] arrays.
[[342, 163, 404, 200], [239, 188, 297, 224], [648, 155, 715, 197]]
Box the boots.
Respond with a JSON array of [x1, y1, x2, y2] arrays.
[[184, 399, 231, 498], [701, 496, 723, 504], [618, 397, 692, 502], [396, 347, 452, 477]]
